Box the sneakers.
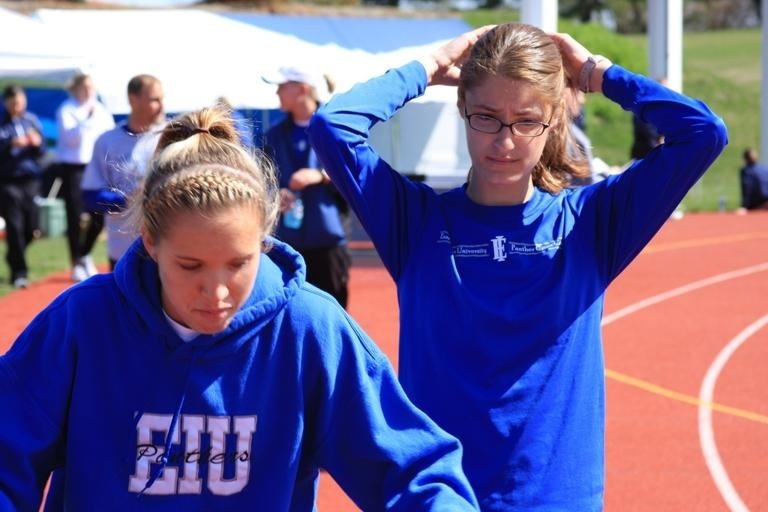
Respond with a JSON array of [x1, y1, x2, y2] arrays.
[[13, 277, 29, 289], [71, 253, 99, 282]]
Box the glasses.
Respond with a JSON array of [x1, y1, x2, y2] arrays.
[[459, 90, 556, 138]]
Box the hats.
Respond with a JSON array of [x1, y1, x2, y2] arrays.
[[260, 60, 324, 89]]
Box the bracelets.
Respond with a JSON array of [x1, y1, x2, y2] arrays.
[[577, 54, 610, 94]]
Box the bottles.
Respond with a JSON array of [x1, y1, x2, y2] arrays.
[[282, 184, 305, 230]]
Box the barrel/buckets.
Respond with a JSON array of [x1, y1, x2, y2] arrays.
[[31, 195, 70, 237]]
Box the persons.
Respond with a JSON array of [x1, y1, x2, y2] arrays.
[[304, 22, 727, 512], [78, 74, 169, 274], [0, 102, 481, 512], [735, 147, 768, 216], [0, 83, 48, 286], [261, 64, 351, 310], [53, 75, 116, 282], [564, 77, 670, 195]]
